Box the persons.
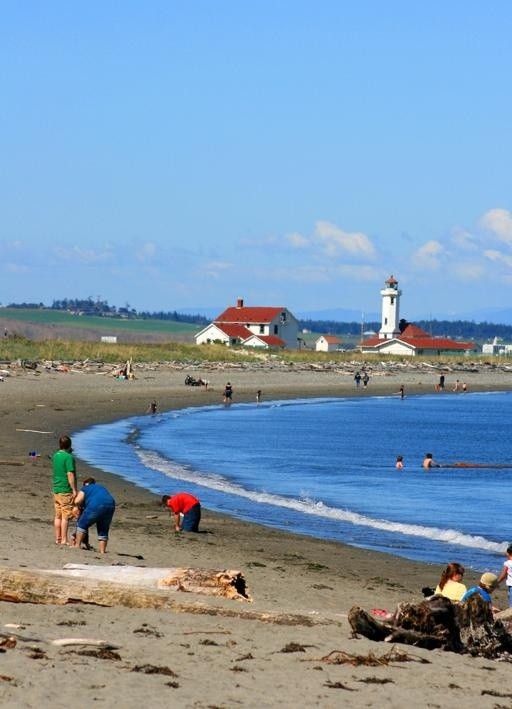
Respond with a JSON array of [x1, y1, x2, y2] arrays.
[[459, 572, 499, 609], [255, 390, 262, 404], [395, 456, 403, 469], [423, 453, 438, 469], [399, 384, 405, 400], [113, 369, 126, 383], [223, 381, 233, 403], [161, 492, 201, 533], [50, 434, 79, 546], [149, 399, 157, 415], [434, 384, 441, 392], [433, 562, 467, 602], [362, 372, 369, 389], [462, 382, 467, 392], [439, 372, 444, 388], [353, 371, 361, 388], [69, 476, 117, 555], [452, 379, 460, 391], [69, 490, 93, 552], [496, 544, 511, 607]]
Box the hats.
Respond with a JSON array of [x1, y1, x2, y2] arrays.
[[481, 572, 500, 588]]
